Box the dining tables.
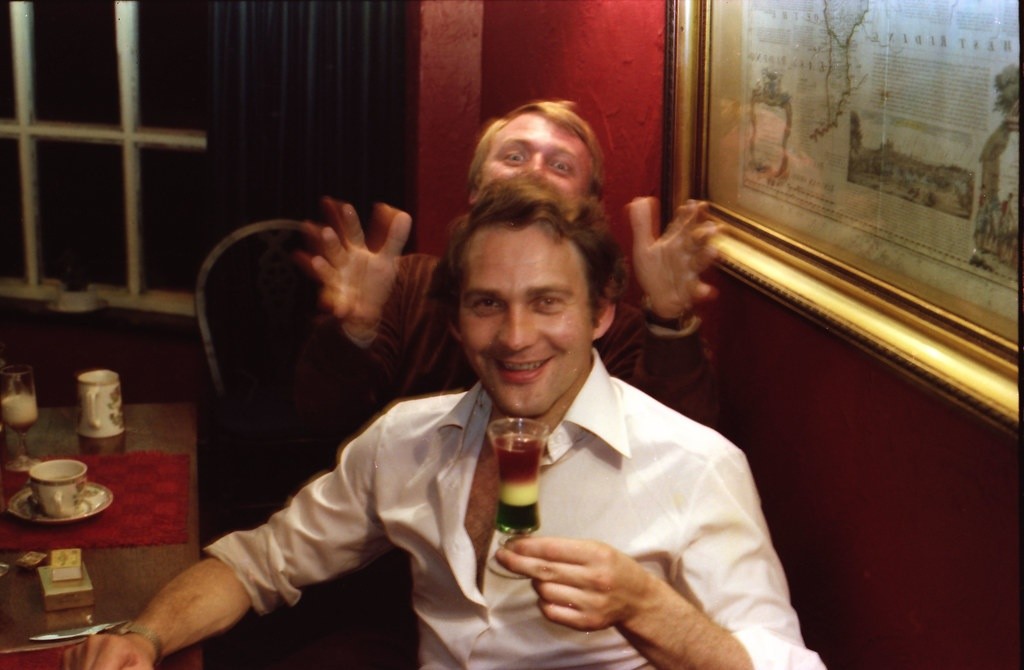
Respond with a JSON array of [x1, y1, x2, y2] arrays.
[[0, 401, 205, 670]]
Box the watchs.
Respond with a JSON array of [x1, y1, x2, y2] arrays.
[[644, 305, 692, 331], [96, 619, 163, 666]]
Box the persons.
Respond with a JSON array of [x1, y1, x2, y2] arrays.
[[58, 98, 831, 670]]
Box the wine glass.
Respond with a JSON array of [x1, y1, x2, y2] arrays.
[[487, 418, 549, 579], [0, 364, 42, 472]]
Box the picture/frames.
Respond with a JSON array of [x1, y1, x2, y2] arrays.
[[659, 0, 1024, 453]]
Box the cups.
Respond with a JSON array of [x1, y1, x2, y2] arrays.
[[29, 459, 87, 519], [76, 369, 125, 438]]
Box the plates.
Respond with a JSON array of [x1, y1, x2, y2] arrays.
[[6, 481, 113, 525]]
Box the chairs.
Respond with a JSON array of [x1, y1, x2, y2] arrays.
[[192, 217, 348, 561]]
